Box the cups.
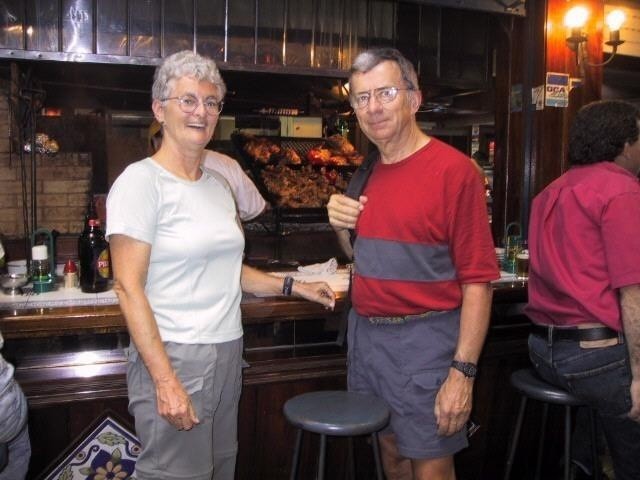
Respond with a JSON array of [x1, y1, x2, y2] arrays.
[[515, 254, 529, 278], [6, 260, 28, 277], [495, 248, 506, 266]]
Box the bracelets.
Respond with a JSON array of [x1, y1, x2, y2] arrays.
[[282, 277, 293, 297]]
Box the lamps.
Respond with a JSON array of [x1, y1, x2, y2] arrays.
[[563, 7, 625, 69]]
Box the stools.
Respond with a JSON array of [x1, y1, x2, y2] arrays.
[[285, 390, 393, 479], [505, 368, 600, 480]]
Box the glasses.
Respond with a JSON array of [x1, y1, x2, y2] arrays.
[[159, 94, 225, 116], [348, 85, 413, 110]]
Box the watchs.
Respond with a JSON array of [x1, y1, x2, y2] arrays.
[[451, 360, 477, 378]]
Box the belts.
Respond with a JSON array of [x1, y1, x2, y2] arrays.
[[528, 322, 617, 342]]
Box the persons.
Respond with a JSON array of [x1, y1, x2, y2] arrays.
[[326, 46, 500, 479], [528, 98, 640, 474], [104, 50, 338, 479]]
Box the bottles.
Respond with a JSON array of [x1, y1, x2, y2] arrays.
[[30, 245, 51, 283], [62, 258, 78, 290], [80, 219, 109, 294]]
